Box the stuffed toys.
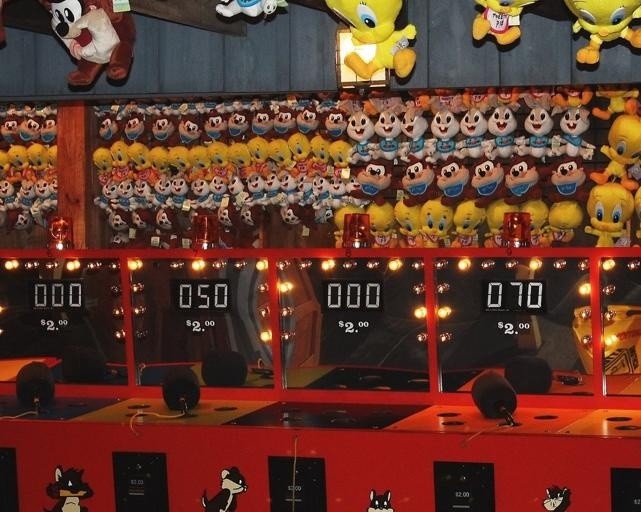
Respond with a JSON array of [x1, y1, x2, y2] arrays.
[[324, 0, 418, 80], [94, 85, 641, 251], [1, 101, 59, 232], [87, 83, 641, 251], [563, 0, 641, 65], [40, 0, 138, 87], [472, 0, 537, 47]]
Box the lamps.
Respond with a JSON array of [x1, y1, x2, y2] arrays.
[[335, 24, 391, 89]]
